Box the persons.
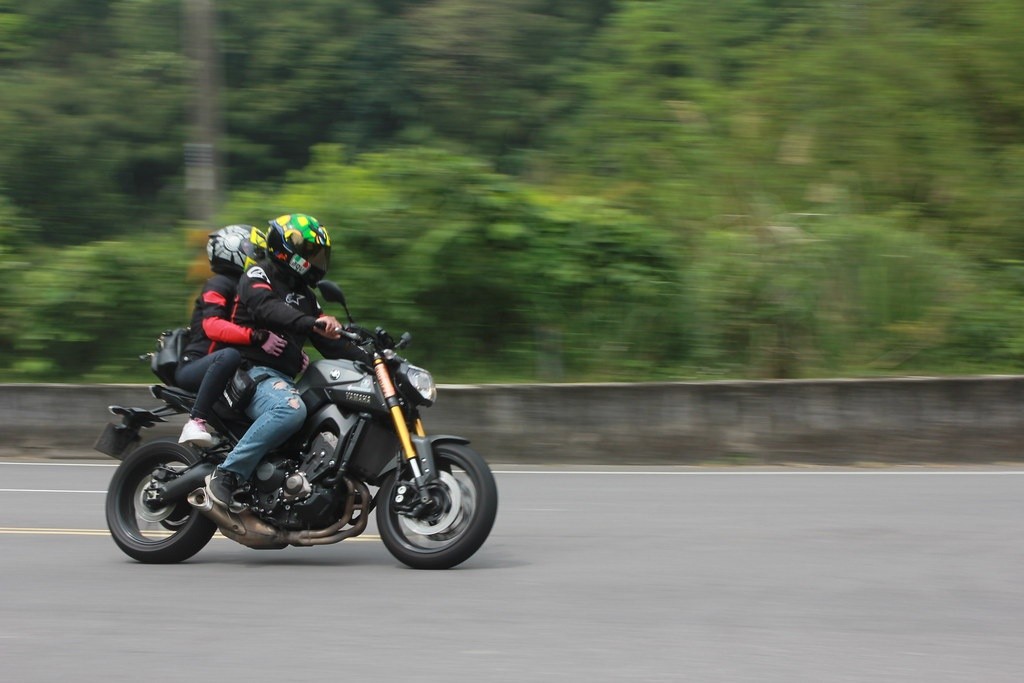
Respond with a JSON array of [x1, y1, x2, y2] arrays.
[[173, 211, 355, 510]]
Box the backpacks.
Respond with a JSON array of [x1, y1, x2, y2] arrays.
[[156, 328, 189, 369]]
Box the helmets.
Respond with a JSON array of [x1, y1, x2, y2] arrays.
[[207, 224, 266, 278], [266, 214, 331, 289]]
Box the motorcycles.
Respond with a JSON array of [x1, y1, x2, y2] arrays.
[[93, 280, 499, 570]]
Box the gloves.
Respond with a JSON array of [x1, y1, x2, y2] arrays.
[[300, 352, 309, 373], [252, 330, 287, 357]]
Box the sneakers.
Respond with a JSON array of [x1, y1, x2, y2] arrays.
[[178, 420, 212, 448], [205, 469, 234, 510]]
